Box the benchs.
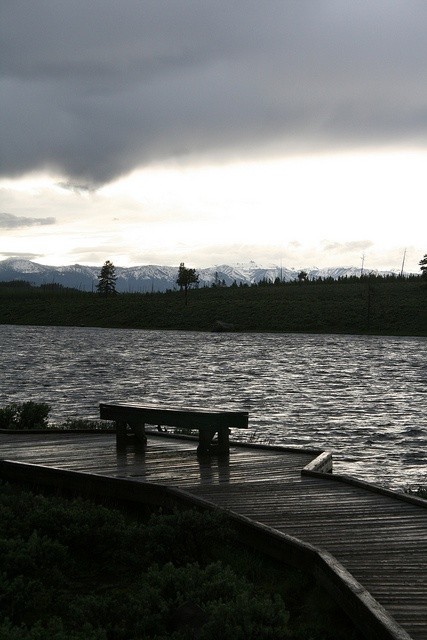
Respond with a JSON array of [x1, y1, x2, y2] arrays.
[[101, 396, 250, 461]]
